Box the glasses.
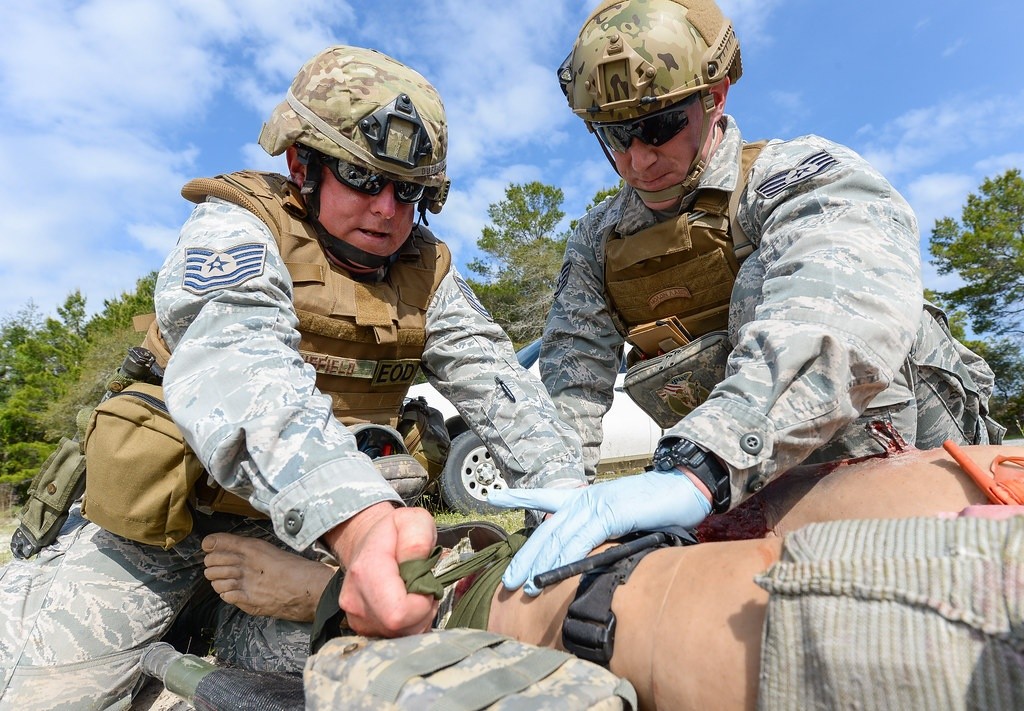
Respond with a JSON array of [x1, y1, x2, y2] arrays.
[[294, 141, 431, 204], [592, 90, 700, 152]]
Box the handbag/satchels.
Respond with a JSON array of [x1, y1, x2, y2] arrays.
[[80, 380, 203, 552], [347, 423, 430, 508], [622, 329, 733, 428]]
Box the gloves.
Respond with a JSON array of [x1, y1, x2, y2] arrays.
[[486, 468, 713, 598]]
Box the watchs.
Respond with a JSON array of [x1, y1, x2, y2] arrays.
[[653, 439, 731, 514]]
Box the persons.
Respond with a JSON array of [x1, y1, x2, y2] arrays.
[[202, 444, 1024, 711], [0, 43, 588, 711], [487, 0, 1006, 597]]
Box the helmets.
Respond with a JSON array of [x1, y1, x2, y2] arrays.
[[257, 45, 451, 214], [557, 0, 744, 134]]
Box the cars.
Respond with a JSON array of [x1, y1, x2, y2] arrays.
[[395, 322, 676, 524]]
